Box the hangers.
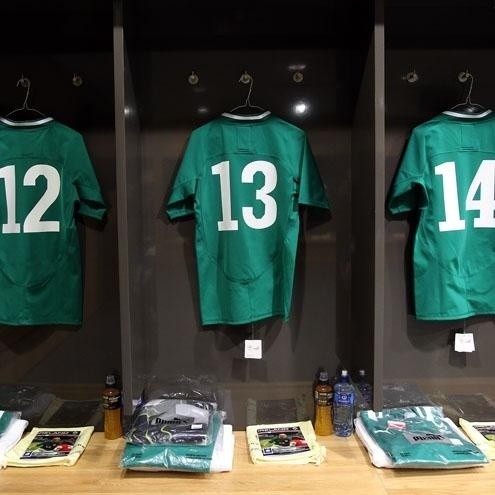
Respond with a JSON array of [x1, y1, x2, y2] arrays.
[[227, 75, 266, 115], [447, 74, 489, 113], [3, 79, 44, 120]]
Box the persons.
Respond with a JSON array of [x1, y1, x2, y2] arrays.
[[52, 440, 73, 453], [264, 433, 290, 448], [27, 437, 61, 452], [289, 436, 308, 447]]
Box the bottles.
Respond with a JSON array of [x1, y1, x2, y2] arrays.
[[315, 369, 371, 437], [101, 375, 123, 440]]
